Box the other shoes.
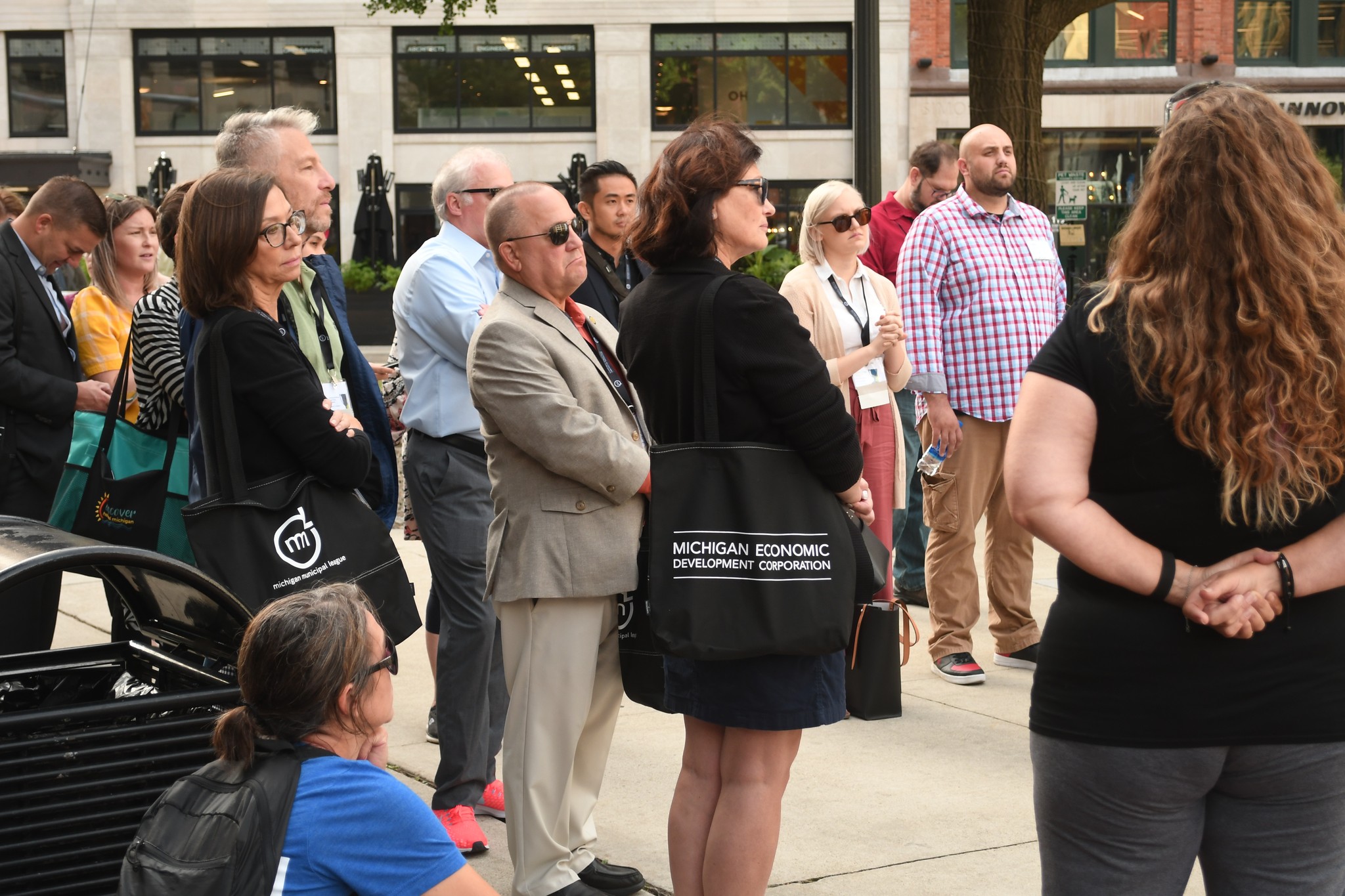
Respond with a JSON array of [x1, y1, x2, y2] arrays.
[[426, 706, 441, 744], [892, 581, 929, 608]]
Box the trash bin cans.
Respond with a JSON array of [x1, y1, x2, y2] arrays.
[[0, 516, 256, 896]]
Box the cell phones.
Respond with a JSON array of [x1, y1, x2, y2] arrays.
[[119, 390, 138, 402]]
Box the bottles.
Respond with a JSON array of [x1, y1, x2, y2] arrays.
[[917, 419, 963, 476]]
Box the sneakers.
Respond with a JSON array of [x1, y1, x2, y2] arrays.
[[475, 780, 506, 819], [931, 652, 985, 684], [994, 641, 1040, 671], [428, 807, 490, 855]]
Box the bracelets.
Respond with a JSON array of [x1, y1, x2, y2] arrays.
[[1269, 549, 1295, 610], [1147, 546, 1176, 601], [1184, 564, 1198, 632]]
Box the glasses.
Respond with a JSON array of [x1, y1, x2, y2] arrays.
[[1162, 79, 1256, 132], [343, 637, 399, 677], [104, 193, 135, 203], [913, 165, 956, 199], [810, 205, 872, 232], [259, 209, 307, 248], [455, 188, 501, 198], [506, 217, 584, 246], [732, 178, 769, 205]]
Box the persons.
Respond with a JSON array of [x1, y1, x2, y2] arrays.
[[466, 183, 650, 896], [616, 111, 874, 896], [212, 581, 502, 896], [569, 160, 654, 333], [1004, 80, 1345, 896], [389, 151, 516, 856], [778, 124, 1067, 686], [0, 105, 441, 743]]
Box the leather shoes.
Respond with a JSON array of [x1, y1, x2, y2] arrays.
[[576, 857, 645, 891], [544, 880, 610, 896]]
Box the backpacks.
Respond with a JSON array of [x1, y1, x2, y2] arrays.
[[116, 736, 341, 896]]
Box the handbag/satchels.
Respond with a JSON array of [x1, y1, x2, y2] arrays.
[[640, 444, 857, 660], [840, 498, 891, 602], [182, 467, 421, 645], [615, 495, 670, 712], [46, 410, 194, 579], [848, 597, 916, 721]]
